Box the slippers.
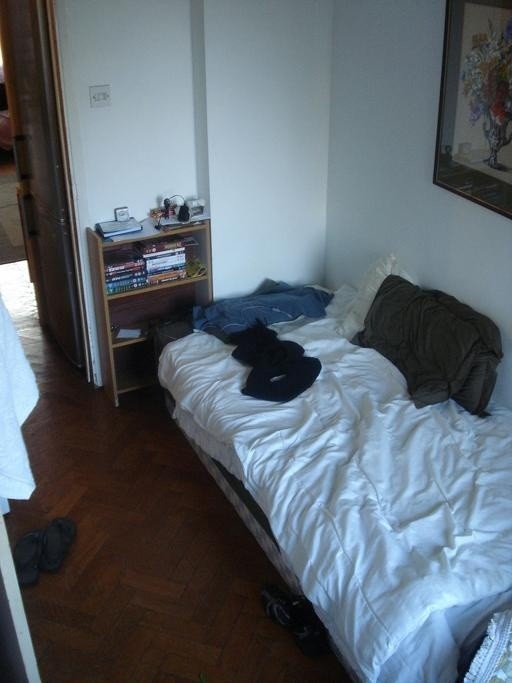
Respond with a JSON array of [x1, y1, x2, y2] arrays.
[[13, 528, 48, 586], [40, 517, 77, 572], [261, 585, 329, 658]]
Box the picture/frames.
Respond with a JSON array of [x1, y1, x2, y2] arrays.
[[433, 1, 512, 221]]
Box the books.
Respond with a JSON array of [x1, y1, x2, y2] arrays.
[[96, 217, 143, 237], [149, 205, 210, 232], [103, 233, 187, 296]]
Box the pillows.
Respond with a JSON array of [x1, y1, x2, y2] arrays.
[[335, 250, 501, 414]]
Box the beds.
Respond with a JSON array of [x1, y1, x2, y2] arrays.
[[156, 281, 512, 683]]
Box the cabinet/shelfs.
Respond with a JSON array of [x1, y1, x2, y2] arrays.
[[86, 215, 213, 407]]
